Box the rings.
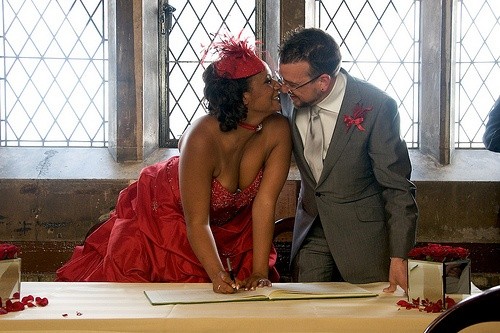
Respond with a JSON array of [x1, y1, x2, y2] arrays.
[[217, 285, 220, 290], [259, 280, 265, 282]]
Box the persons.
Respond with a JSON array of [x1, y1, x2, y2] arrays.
[[274, 28, 419, 295], [53, 28, 292, 294]]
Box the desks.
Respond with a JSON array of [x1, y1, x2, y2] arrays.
[[0, 281, 500, 333]]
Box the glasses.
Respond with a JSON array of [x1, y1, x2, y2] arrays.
[[275, 70, 333, 95]]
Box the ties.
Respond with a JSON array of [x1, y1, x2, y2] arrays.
[[304, 106, 323, 184]]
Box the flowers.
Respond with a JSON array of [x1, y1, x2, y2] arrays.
[[0, 242, 21, 260], [407, 244, 470, 275]]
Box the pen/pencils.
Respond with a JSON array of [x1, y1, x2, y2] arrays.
[[227, 258, 235, 292]]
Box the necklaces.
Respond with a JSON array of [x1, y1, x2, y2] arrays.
[[238, 123, 263, 132]]
